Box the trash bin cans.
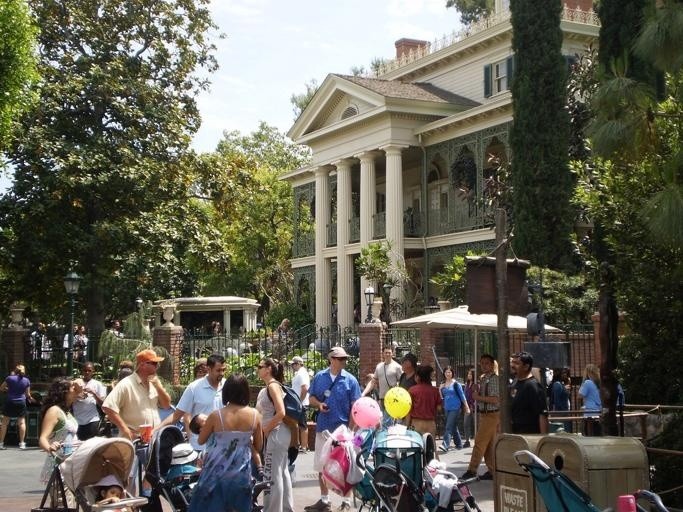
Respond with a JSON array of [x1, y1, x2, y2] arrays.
[[492, 430, 652, 511]]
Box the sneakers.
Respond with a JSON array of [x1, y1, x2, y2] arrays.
[[0, 442, 25, 450], [457, 470, 478, 481], [336, 502, 351, 512], [304, 498, 331, 512], [438, 440, 471, 452], [479, 472, 493, 481]]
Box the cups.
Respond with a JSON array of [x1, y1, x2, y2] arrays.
[[138, 424, 153, 446]]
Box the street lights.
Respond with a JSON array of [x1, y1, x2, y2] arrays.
[[363, 282, 375, 323], [383, 282, 392, 333], [60, 269, 84, 378]]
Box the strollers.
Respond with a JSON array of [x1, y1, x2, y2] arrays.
[[511, 449, 611, 512], [48, 436, 149, 512], [319, 425, 483, 512], [631, 489, 669, 512], [133, 425, 271, 512]]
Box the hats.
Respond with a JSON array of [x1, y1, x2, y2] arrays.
[[85, 474, 126, 505], [287, 355, 304, 365], [401, 353, 419, 361], [328, 346, 350, 362], [135, 349, 166, 363], [170, 442, 199, 465]]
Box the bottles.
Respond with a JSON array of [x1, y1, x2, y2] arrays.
[[63, 431, 72, 455], [615, 494, 635, 511], [581, 417, 600, 435]]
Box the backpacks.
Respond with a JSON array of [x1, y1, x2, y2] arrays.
[[267, 380, 303, 427], [319, 440, 353, 497]]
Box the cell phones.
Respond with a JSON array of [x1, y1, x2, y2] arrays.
[[323, 405, 329, 409]]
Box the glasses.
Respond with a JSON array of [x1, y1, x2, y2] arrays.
[[258, 365, 268, 369]]
